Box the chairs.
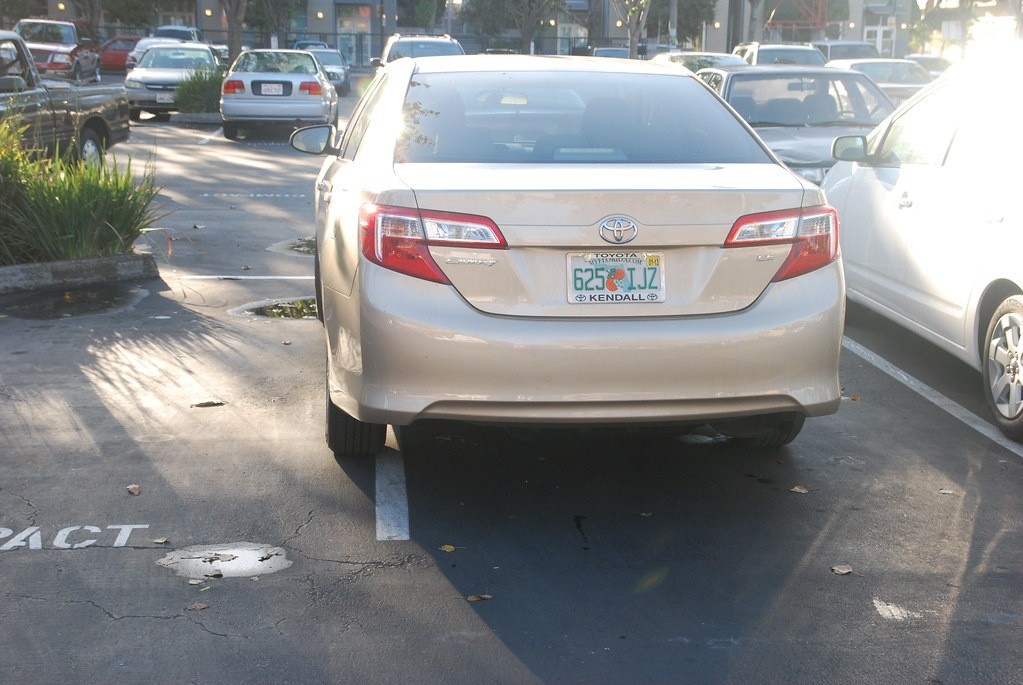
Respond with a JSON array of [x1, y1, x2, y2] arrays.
[[730, 91, 839, 125]]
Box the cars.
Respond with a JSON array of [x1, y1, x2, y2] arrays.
[[695, 65, 899, 189], [96, 36, 143, 72], [293, 40, 328, 52], [807, 56, 935, 126], [648, 52, 750, 77], [289, 55, 849, 455], [219, 49, 338, 139], [123, 44, 228, 121], [301, 48, 352, 96], [125, 36, 186, 75], [905, 54, 951, 77], [0, 20, 102, 86]]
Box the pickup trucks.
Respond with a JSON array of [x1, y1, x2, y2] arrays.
[[0, 31, 129, 176]]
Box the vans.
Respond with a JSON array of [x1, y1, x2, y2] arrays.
[[816, 67, 1023, 444]]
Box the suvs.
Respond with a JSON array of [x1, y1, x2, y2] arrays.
[[807, 42, 882, 81], [572, 45, 640, 62], [731, 42, 829, 99], [368, 32, 466, 82], [150, 26, 206, 47]]
